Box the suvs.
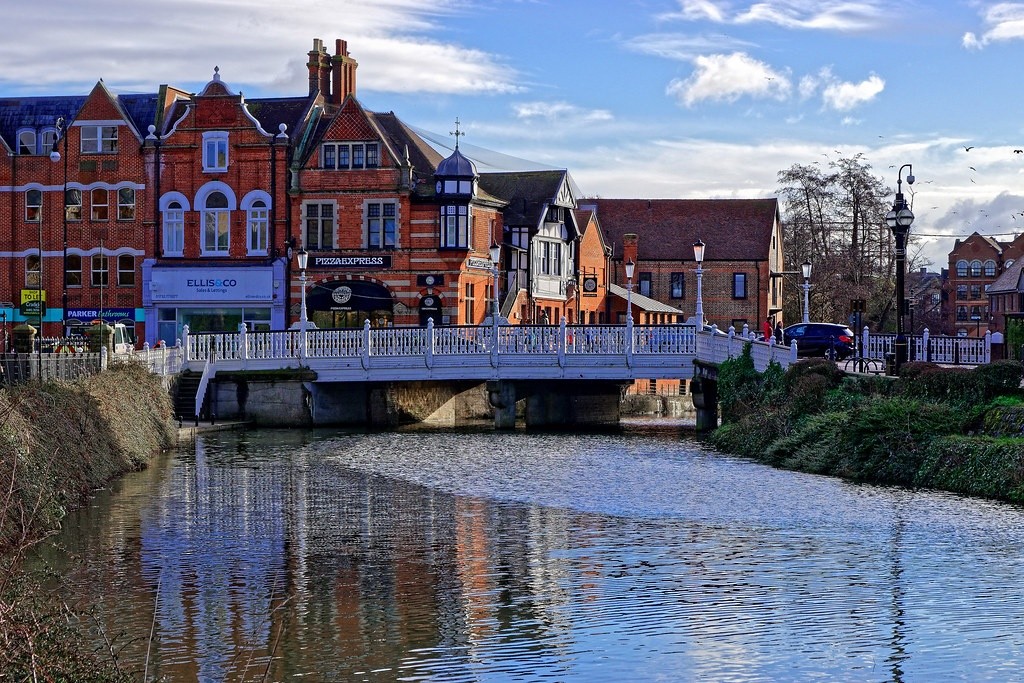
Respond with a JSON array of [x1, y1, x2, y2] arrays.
[[757, 322, 854, 362]]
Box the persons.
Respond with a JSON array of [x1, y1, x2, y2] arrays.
[[763, 316, 784, 345]]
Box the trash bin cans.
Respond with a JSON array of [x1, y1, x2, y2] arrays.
[[886, 353, 896, 375]]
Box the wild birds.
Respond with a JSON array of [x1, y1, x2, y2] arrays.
[[930, 206, 1024, 249], [860, 157, 870, 161], [813, 150, 842, 165], [1014, 149, 1023, 155], [915, 179, 934, 186], [889, 164, 897, 169], [764, 76, 775, 81], [962, 145, 974, 152], [878, 135, 885, 139], [969, 165, 977, 172], [970, 177, 977, 184]]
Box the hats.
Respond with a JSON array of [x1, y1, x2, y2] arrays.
[[767, 317, 774, 320]]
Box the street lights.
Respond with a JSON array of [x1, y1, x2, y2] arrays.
[[884, 163, 915, 374], [692, 238, 706, 330], [297, 246, 312, 330], [624, 257, 636, 322], [800, 258, 812, 321]]
